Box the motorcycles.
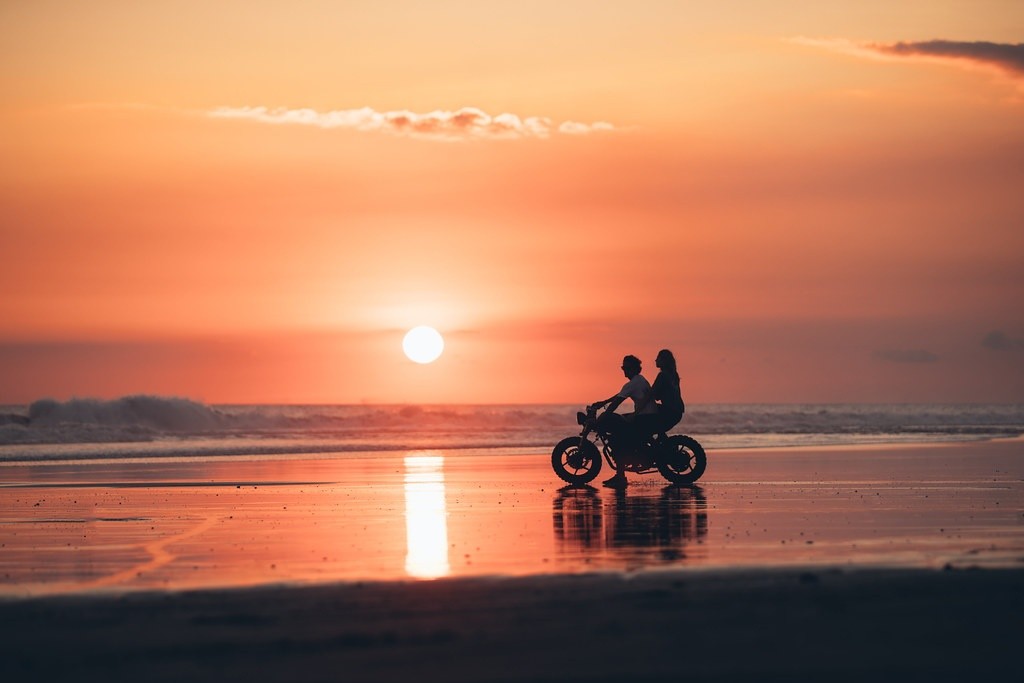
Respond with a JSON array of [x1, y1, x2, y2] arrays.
[[551, 403, 708, 487]]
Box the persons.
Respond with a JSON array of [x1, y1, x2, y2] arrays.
[[592, 349, 685, 490]]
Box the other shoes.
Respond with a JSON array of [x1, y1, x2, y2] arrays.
[[602, 474, 628, 487]]
